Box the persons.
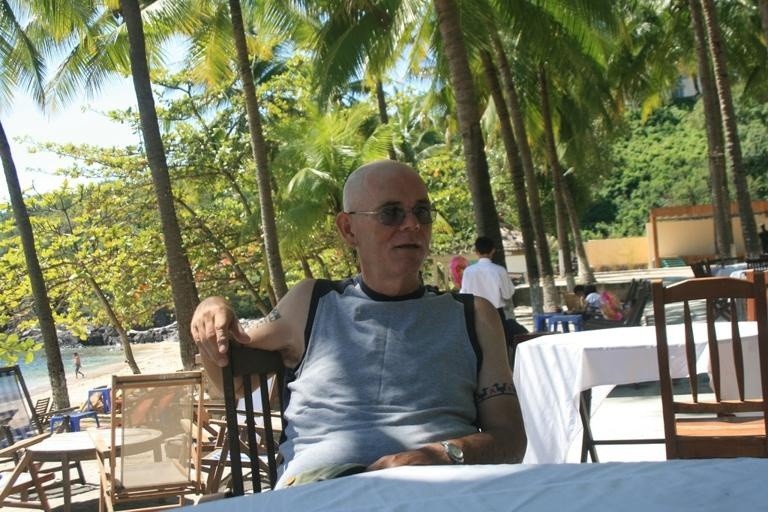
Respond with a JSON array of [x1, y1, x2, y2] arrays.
[[572, 284, 585, 313], [457, 236, 516, 354], [189, 159, 528, 496], [450, 255, 470, 293], [758, 223, 768, 256], [72, 352, 86, 379], [583, 284, 604, 321]]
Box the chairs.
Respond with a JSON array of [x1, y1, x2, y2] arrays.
[[649, 268, 767, 461], [685, 257, 768, 322], [534, 275, 652, 330], [1, 352, 293, 511]]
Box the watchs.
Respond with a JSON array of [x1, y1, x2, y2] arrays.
[[439, 440, 466, 466]]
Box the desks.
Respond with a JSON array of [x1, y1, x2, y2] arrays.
[[510, 320, 768, 465], [157, 455, 768, 512]]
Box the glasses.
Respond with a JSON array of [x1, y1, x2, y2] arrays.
[[345, 201, 439, 227]]
